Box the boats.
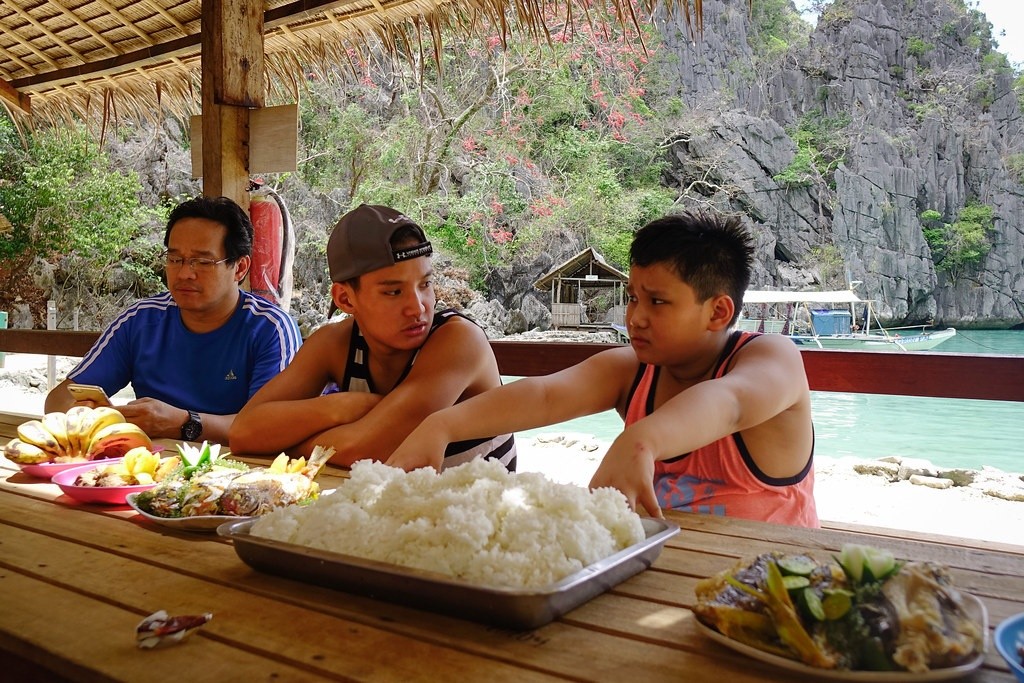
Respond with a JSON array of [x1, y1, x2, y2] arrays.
[[734, 279, 958, 354]]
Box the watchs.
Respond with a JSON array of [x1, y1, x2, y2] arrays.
[[180, 411, 203, 440]]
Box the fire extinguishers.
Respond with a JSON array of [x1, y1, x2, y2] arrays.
[[246, 178, 289, 307]]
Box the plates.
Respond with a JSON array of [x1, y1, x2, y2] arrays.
[[124, 481, 335, 529], [51, 462, 155, 505], [693, 591, 986, 678], [996, 614, 1024, 679], [20, 444, 165, 478]]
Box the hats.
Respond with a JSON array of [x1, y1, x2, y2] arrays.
[[324, 202, 436, 315]]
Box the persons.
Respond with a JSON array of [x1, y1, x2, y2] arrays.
[[384, 209, 820, 530], [44, 194, 303, 448], [229, 203, 516, 475]]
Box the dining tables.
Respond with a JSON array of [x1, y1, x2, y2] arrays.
[[0, 414, 1024, 682]]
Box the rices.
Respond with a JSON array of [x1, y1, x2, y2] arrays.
[[249, 453, 646, 590]]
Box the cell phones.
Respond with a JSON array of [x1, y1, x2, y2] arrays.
[[66, 383, 113, 407]]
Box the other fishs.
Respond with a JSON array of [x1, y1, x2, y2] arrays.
[[137, 443, 336, 515]]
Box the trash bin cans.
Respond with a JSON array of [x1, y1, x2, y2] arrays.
[[811, 308, 851, 336]]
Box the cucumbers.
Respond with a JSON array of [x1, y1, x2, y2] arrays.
[[777, 557, 852, 623]]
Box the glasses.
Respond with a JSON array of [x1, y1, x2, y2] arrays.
[[157, 249, 239, 272]]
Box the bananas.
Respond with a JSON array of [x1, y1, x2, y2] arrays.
[[3, 405, 154, 463]]
